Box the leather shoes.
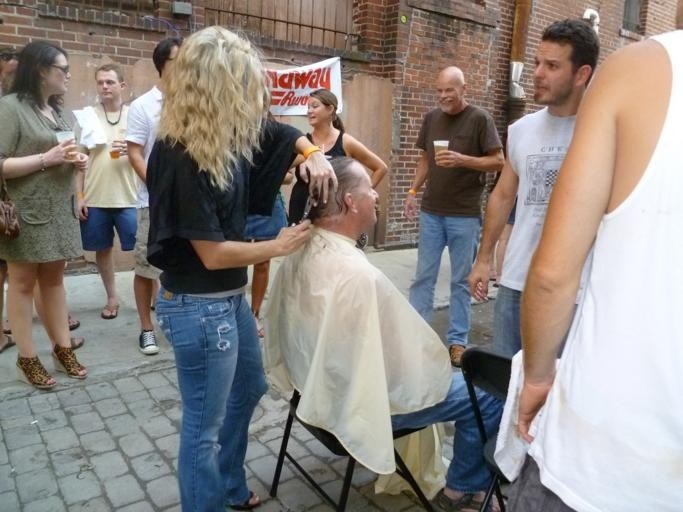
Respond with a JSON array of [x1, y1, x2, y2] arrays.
[[451, 345, 465, 366]]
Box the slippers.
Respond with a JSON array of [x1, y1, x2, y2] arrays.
[[0, 335, 15, 353], [101, 303, 119, 319], [230, 490, 261, 510], [70, 336, 85, 350]]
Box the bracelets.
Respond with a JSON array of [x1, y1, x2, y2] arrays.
[[409, 188, 416, 196]]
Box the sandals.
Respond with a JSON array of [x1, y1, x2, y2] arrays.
[[429, 488, 507, 512]]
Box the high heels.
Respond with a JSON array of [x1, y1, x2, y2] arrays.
[[16, 356, 56, 389], [52, 344, 88, 378]]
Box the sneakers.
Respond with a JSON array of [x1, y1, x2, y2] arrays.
[[139, 329, 158, 354]]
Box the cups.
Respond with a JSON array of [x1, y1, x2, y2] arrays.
[[108, 137, 121, 160], [56, 131, 76, 160], [433, 140, 449, 166]]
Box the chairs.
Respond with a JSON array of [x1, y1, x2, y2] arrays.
[[270, 345, 434, 511], [461, 348, 524, 511]]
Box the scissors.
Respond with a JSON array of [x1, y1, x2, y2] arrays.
[[288, 194, 315, 228]]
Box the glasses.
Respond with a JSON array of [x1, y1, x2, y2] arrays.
[[50, 64, 69, 73]]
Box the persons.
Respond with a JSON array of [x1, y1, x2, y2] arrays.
[[147, 18, 339, 512], [464, 17, 600, 360], [243, 90, 389, 339], [0, 36, 184, 390], [267, 158, 506, 511], [503, 29, 682, 510], [400, 65, 505, 369]]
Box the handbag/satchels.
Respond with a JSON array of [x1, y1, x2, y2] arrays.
[[0, 158, 20, 239]]
